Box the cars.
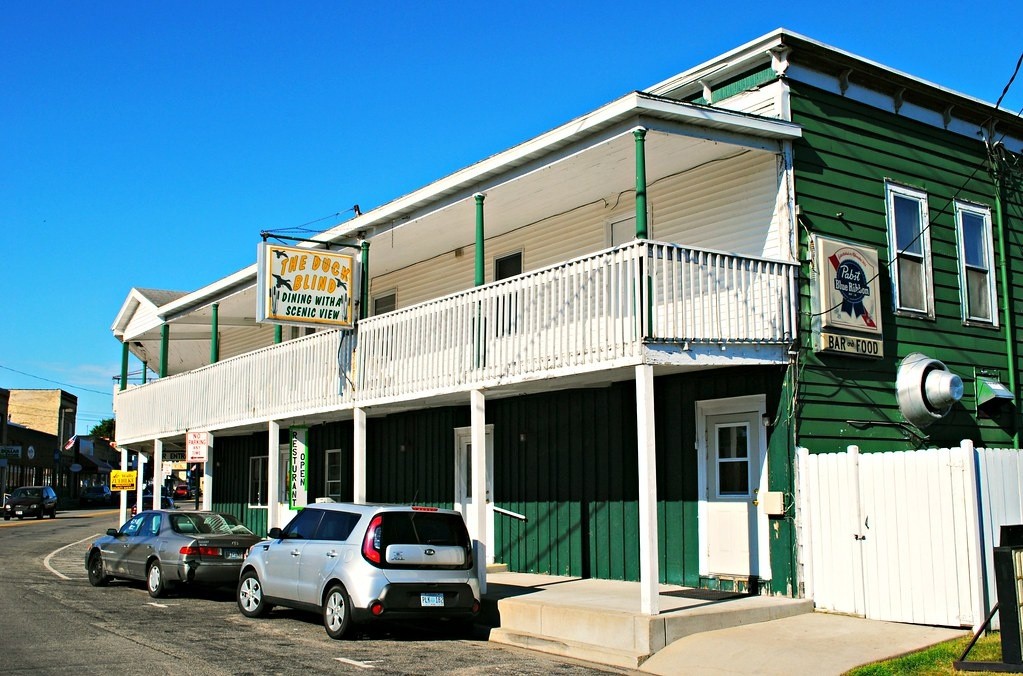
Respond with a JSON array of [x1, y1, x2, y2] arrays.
[[80, 485, 112, 509], [84, 508, 270, 599], [112, 475, 201, 506], [237, 502, 482, 641], [131, 495, 180, 520], [4, 485, 58, 521]]
